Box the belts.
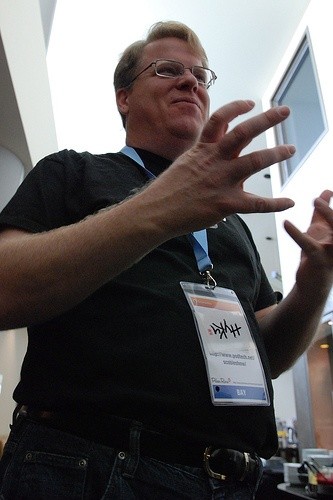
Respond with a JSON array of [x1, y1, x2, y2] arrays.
[[16, 403, 257, 485]]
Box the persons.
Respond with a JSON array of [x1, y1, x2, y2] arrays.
[[0, 20, 333, 500]]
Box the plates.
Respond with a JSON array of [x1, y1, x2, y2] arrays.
[[302, 447, 333, 466]]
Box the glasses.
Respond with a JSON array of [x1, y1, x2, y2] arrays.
[[125, 59, 217, 89]]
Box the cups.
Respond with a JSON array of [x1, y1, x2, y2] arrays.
[[308, 464, 317, 492], [318, 469, 333, 495], [282, 464, 302, 483]]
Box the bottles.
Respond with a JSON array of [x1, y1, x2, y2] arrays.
[[286, 427, 299, 463], [278, 435, 285, 456]]
